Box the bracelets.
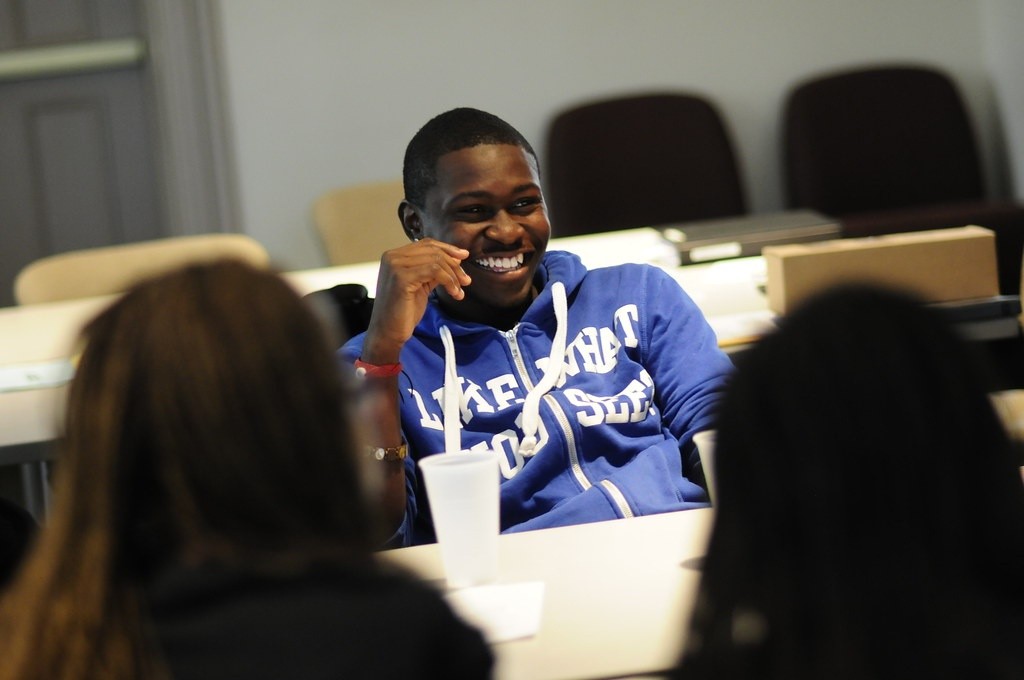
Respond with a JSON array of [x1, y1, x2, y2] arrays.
[[356, 436, 410, 460], [355, 359, 403, 380]]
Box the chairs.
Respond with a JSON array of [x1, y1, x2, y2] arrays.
[[540, 91, 756, 241], [311, 180, 425, 268], [13, 233, 269, 530], [780, 62, 1024, 300]]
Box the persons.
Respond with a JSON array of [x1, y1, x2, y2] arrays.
[[338, 107, 739, 553], [657, 281, 1023, 680], [1, 258, 494, 680]]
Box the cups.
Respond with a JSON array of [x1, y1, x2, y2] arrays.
[[418, 450, 502, 589]]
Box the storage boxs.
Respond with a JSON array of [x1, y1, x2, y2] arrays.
[[762, 221, 1000, 322]]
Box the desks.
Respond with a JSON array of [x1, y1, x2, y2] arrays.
[[0, 229, 777, 475], [370, 504, 717, 680]]
